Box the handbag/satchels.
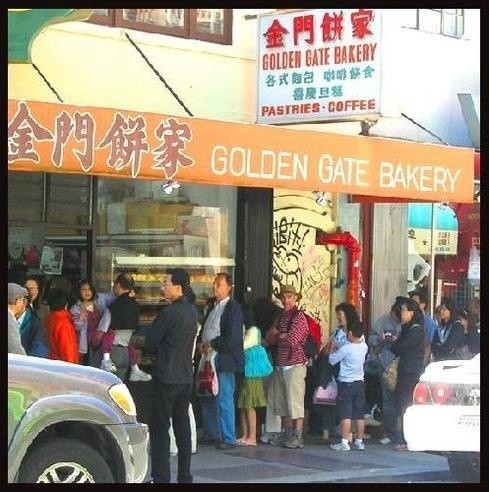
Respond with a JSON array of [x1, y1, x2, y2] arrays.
[[312, 378, 338, 407], [244, 344, 273, 379], [194, 350, 219, 397], [363, 342, 396, 374], [451, 344, 473, 360]]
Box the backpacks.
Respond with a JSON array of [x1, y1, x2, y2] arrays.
[[277, 310, 321, 358]]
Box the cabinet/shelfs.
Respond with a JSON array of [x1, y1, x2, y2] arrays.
[[102, 250, 237, 329]]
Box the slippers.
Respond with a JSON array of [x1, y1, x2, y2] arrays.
[[238, 441, 257, 446]]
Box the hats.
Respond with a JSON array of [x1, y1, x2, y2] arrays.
[[7, 283, 30, 301]]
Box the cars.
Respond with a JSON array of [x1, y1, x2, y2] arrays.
[[401, 349, 480, 482], [7, 348, 153, 487]]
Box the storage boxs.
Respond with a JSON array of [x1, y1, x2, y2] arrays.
[[95, 197, 201, 234]]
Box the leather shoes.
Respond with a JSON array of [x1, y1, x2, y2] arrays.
[[197, 435, 217, 445], [216, 442, 235, 451]]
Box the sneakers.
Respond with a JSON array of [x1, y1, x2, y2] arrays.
[[130, 368, 151, 382], [329, 431, 408, 452], [257, 430, 305, 449], [100, 360, 118, 373]]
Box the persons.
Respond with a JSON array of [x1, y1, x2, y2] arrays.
[[195, 274, 322, 448], [8, 272, 152, 383], [144, 267, 197, 483], [328, 293, 479, 453]]
